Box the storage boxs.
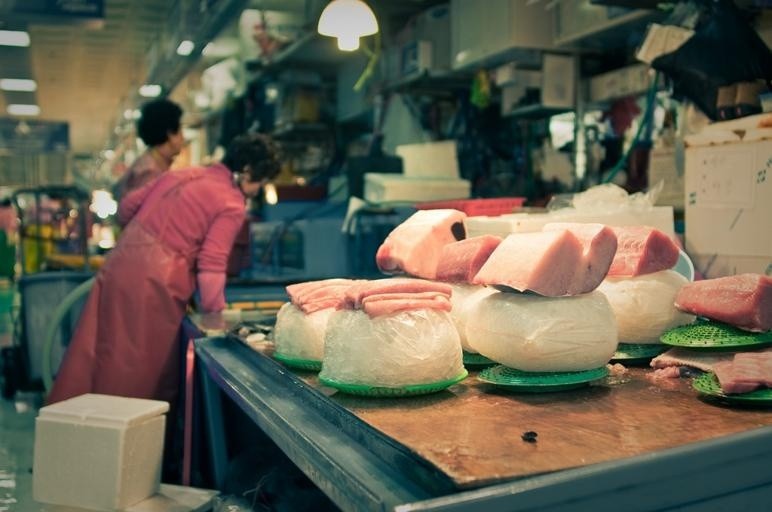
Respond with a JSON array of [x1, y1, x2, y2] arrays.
[[685, 111, 772, 278], [364, 172, 472, 203], [32, 393, 171, 509], [396, 140, 460, 178]]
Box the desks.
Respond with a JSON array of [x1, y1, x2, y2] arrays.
[[195, 331, 771, 511], [181, 288, 291, 339]]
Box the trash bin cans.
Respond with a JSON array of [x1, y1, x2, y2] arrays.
[[19, 271, 94, 383]]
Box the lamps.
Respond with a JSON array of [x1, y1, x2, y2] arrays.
[[318, 0, 379, 50]]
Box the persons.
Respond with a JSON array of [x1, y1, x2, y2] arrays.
[[67, 191, 110, 255], [0, 196, 21, 237], [109, 97, 187, 201], [43, 131, 281, 403]]
